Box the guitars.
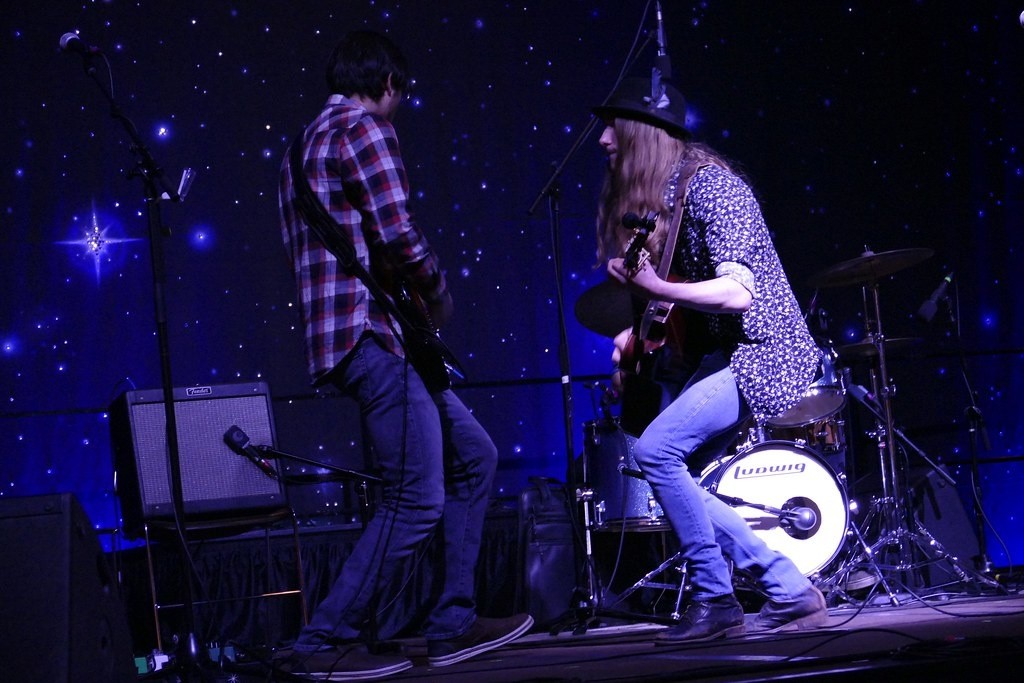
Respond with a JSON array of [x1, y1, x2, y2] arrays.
[[394, 275, 452, 394], [618, 211, 695, 439]]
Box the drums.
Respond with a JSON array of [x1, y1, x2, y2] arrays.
[[767, 334, 848, 429], [581, 420, 673, 533], [769, 413, 848, 455], [697, 439, 849, 578]]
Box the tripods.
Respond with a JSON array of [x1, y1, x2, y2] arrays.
[[816, 266, 1010, 610]]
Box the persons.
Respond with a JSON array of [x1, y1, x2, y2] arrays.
[[599, 113, 832, 646], [278, 31, 535, 682]]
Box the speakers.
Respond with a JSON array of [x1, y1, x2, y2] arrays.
[[0, 494, 139, 683], [107, 382, 287, 524], [844, 463, 981, 590]]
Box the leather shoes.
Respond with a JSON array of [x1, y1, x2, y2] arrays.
[[742, 586, 830, 634], [651, 594, 745, 646]]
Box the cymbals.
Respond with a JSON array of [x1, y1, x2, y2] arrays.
[[806, 248, 936, 289], [575, 279, 634, 338], [835, 334, 924, 356]]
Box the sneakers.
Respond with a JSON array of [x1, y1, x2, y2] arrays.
[[428, 612, 533, 666], [287, 644, 413, 682]]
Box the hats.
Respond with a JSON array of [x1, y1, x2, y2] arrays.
[[592, 81, 691, 140]]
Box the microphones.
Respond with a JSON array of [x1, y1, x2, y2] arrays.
[[60, 33, 103, 58], [917, 273, 953, 321], [841, 367, 875, 401], [222, 425, 279, 478], [781, 506, 816, 530], [656, 0, 666, 57]]
